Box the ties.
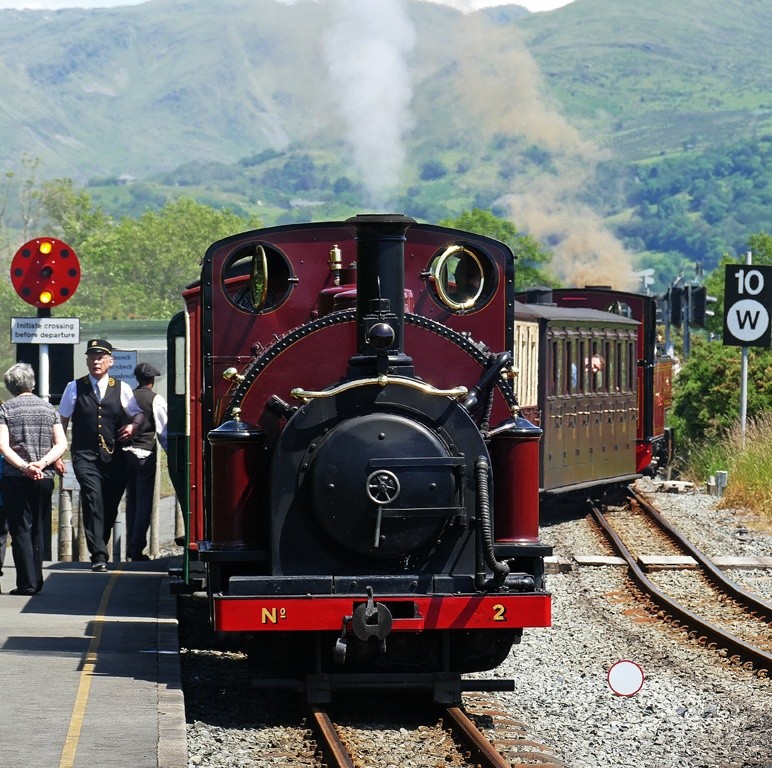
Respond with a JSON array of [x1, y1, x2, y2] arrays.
[[95, 381, 105, 402]]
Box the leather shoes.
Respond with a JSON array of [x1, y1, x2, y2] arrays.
[[92, 561, 108, 572]]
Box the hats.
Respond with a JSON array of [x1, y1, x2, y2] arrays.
[[134, 363, 160, 378], [85, 339, 112, 356]]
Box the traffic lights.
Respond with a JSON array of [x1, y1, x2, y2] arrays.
[[692, 286, 717, 329]]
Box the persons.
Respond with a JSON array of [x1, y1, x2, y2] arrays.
[[58, 339, 145, 571], [0, 362, 68, 596], [125, 362, 168, 563], [570, 354, 607, 388]]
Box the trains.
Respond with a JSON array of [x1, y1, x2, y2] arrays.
[[167, 214, 678, 707]]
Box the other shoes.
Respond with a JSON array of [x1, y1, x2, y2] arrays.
[[10, 588, 36, 595], [132, 554, 150, 561]]
